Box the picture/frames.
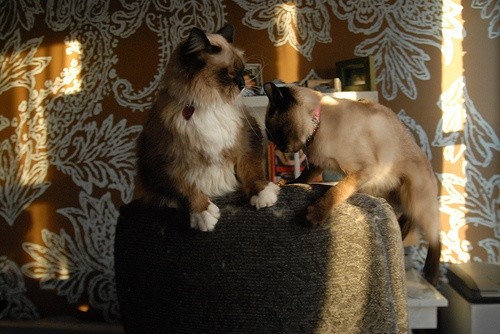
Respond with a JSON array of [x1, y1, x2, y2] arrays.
[[243, 63, 264, 96]]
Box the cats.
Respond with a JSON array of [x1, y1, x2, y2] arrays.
[[264, 81, 445, 279], [134, 28, 277, 234]]
